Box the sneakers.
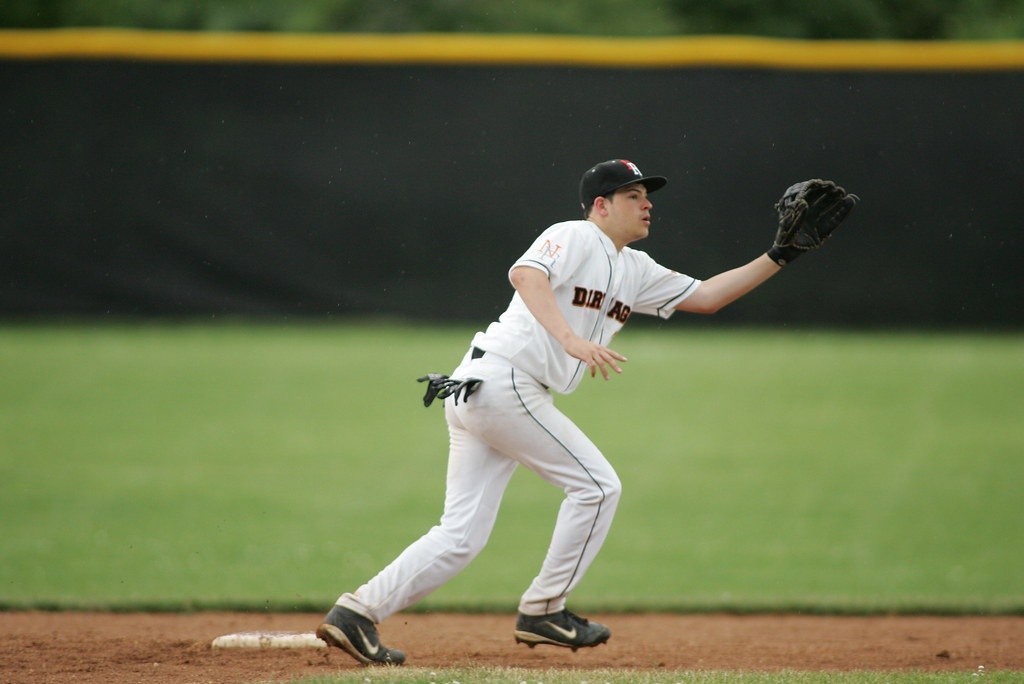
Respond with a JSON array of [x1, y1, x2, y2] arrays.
[[515, 608, 611, 653], [317, 605, 406, 667]]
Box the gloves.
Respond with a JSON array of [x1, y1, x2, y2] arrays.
[[417, 374, 449, 408], [430, 378, 482, 406]]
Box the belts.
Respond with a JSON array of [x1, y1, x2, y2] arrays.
[[471, 347, 549, 389]]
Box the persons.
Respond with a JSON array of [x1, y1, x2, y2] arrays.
[[315, 159, 862, 667]]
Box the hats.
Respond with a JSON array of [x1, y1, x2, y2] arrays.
[[579, 158, 668, 212]]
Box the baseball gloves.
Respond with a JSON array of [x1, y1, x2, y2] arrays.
[[772, 178, 862, 262]]
